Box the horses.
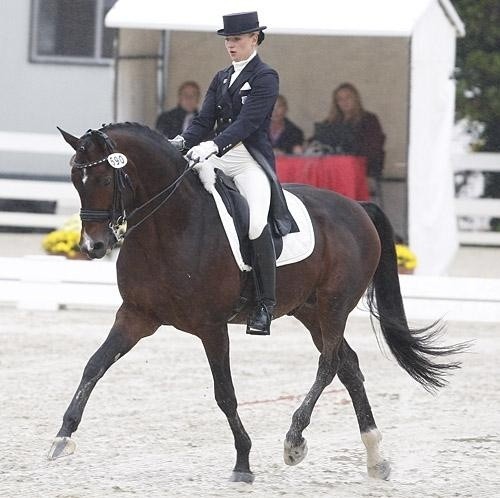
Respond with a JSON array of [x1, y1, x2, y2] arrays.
[[47, 121, 477, 485]]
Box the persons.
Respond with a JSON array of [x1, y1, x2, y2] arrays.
[[168, 10, 301, 334], [154, 79, 203, 154], [314, 83, 386, 195], [268, 95, 306, 157]]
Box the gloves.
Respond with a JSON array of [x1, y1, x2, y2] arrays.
[[185, 140, 219, 164], [168, 134, 186, 150]]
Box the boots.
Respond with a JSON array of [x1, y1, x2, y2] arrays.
[[248, 223, 278, 331]]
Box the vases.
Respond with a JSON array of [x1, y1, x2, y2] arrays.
[[397, 265, 415, 275], [64, 249, 94, 261]]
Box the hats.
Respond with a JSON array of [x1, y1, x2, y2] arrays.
[[216, 10, 267, 37]]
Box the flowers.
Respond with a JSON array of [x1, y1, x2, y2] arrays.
[[395, 243, 420, 269], [40, 229, 82, 259]]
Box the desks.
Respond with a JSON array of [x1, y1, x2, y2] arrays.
[[271, 145, 370, 206]]
